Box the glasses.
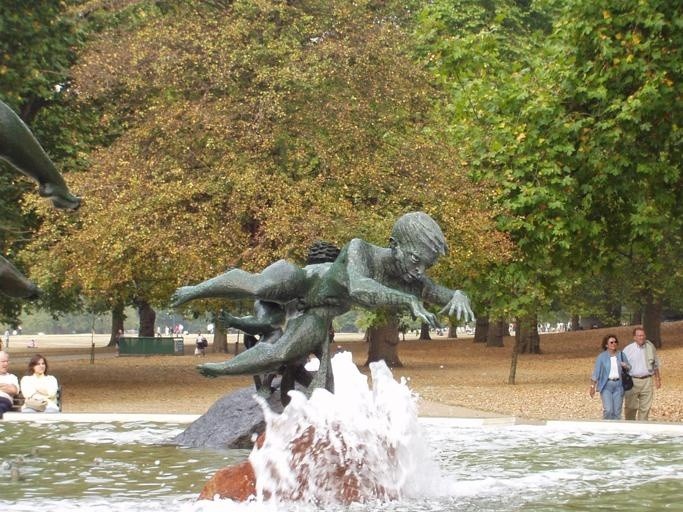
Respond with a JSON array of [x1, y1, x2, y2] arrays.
[[611, 341, 617, 344]]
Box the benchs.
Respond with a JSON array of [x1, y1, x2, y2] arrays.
[[8, 382, 64, 412]]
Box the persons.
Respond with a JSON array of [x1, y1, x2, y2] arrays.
[[0, 350, 59, 419], [5, 329, 9, 348], [157, 323, 184, 337], [28, 338, 38, 348], [115, 329, 122, 356], [590, 328, 661, 420], [538, 320, 562, 332]]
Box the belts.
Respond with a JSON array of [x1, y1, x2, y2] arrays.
[[609, 378, 619, 381], [633, 375, 652, 379]]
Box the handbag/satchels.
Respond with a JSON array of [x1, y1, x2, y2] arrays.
[[25, 392, 48, 412], [621, 352, 634, 391]]
